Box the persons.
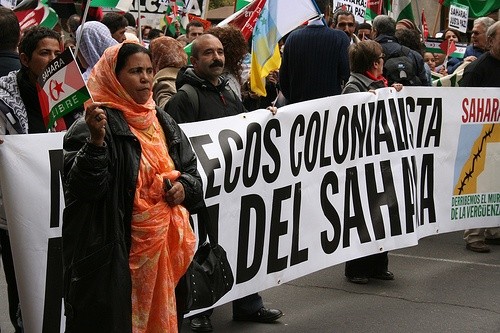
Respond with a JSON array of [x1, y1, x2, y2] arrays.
[[0, 0, 500, 333]]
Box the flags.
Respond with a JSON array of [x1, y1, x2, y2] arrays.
[[164, 0, 186, 37], [394, 1, 414, 26], [365, 0, 382, 22], [183, 0, 265, 71], [87, 0, 133, 13], [34, 46, 91, 132], [418, 8, 430, 41], [17, 0, 60, 50], [437, 0, 500, 21], [250, 0, 328, 97]]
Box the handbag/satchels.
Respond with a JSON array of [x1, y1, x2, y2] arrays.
[[180, 240, 233, 310]]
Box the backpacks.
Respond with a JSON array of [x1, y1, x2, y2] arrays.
[[374, 53, 385, 62], [383, 45, 416, 88]]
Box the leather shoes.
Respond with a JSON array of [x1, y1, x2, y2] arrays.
[[372, 268, 394, 280], [190, 317, 211, 330], [485, 236, 500, 245], [466, 240, 491, 254], [346, 274, 370, 284], [233, 307, 282, 325]]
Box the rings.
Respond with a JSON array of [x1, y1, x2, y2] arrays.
[[101, 124, 106, 127], [94, 116, 102, 122]]
